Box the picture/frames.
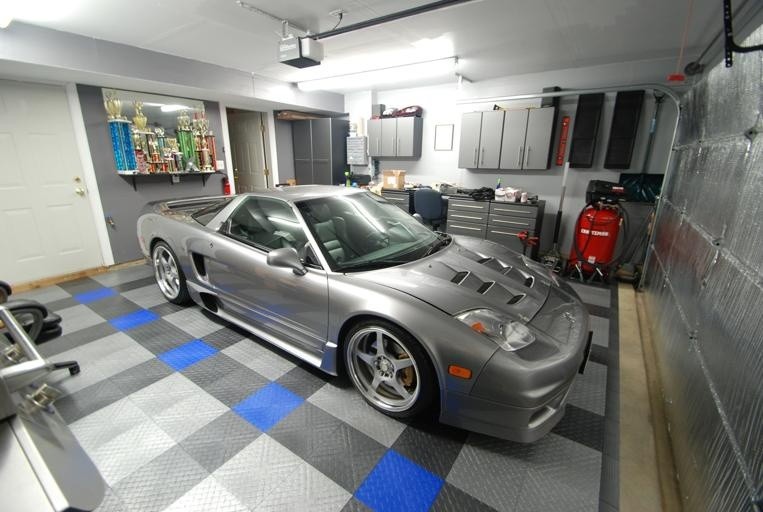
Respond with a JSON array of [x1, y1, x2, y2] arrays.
[[434, 124, 454, 151]]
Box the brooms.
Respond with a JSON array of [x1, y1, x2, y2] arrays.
[[545, 162, 570, 263]]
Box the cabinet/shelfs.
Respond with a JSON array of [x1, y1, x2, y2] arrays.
[[457, 110, 505, 169], [293, 118, 349, 185], [604, 91, 644, 169], [541, 86, 562, 108], [346, 136, 369, 166], [382, 117, 423, 160], [380, 186, 546, 259], [568, 93, 605, 168], [605, 201, 656, 264], [499, 107, 558, 170], [367, 119, 382, 161]]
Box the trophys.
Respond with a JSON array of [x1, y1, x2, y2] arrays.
[[103, 91, 216, 170]]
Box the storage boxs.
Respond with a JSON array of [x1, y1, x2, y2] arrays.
[[383, 169, 406, 190]]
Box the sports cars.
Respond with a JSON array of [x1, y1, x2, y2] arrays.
[[137, 184, 593, 443]]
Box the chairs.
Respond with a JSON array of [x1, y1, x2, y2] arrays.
[[309, 203, 348, 265]]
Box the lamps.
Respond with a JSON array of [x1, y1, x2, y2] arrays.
[[161, 104, 189, 113], [297, 56, 458, 93]]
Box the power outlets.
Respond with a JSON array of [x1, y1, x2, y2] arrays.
[[329, 8, 349, 19]]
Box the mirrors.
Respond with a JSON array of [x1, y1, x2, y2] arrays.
[[101, 88, 206, 140]]
